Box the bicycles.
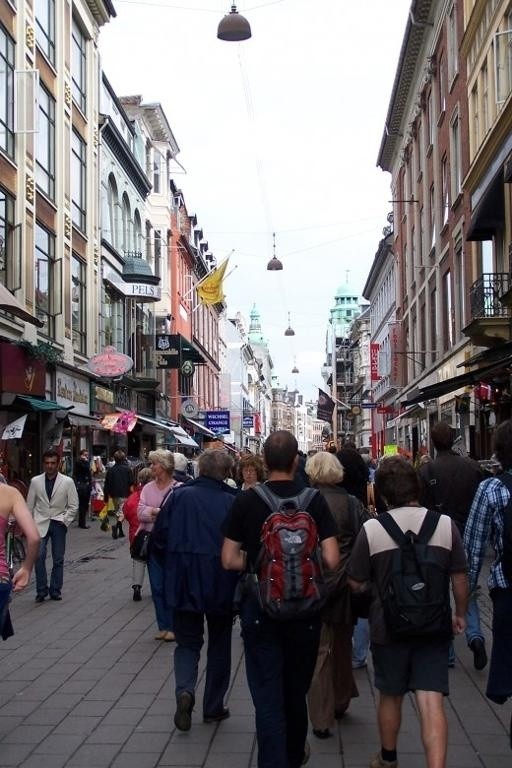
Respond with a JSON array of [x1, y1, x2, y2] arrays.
[[6, 517, 26, 577]]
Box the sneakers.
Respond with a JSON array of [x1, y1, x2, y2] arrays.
[[302, 741, 311, 765], [351, 658, 369, 670], [469, 635, 489, 672], [172, 688, 195, 733], [369, 751, 399, 768], [79, 523, 92, 530], [312, 726, 331, 739], [111, 521, 125, 540], [133, 584, 143, 602], [156, 626, 180, 641], [204, 706, 230, 723], [335, 705, 350, 722]]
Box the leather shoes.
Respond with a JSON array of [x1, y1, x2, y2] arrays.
[[35, 592, 48, 601], [49, 592, 63, 601]]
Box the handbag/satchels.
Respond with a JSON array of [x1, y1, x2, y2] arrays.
[[128, 527, 156, 563]]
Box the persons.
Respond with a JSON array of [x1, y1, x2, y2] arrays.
[[104, 450, 263, 733], [460, 421, 510, 704], [296, 442, 381, 741], [415, 422, 488, 676], [0, 449, 91, 641], [344, 454, 470, 766], [220, 426, 341, 767]]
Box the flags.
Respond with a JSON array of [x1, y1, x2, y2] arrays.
[[387, 322, 404, 387], [316, 388, 335, 424], [195, 256, 231, 306]]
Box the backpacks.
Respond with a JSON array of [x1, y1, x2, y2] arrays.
[[250, 481, 328, 617], [376, 514, 454, 648], [434, 497, 465, 544], [494, 470, 512, 589]]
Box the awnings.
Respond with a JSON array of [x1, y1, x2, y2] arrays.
[[157, 423, 199, 447], [193, 423, 216, 438]]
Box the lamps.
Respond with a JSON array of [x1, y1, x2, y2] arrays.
[[260, 229, 302, 380], [214, 0, 256, 41]]
